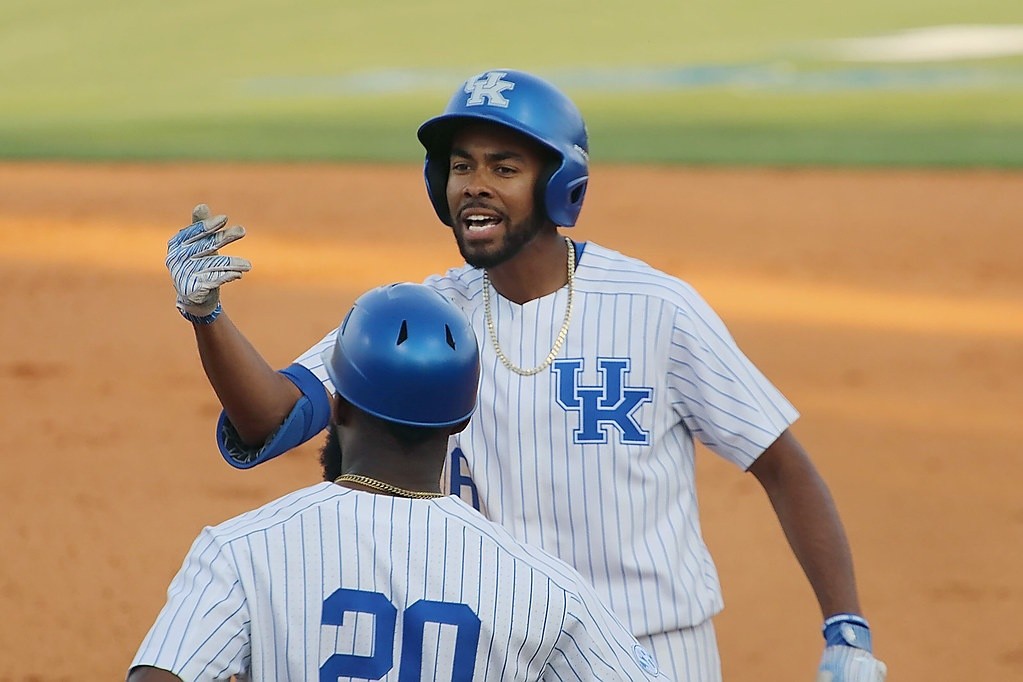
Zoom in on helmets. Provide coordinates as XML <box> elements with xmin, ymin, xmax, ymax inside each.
<box><xmin>319</xmin><ymin>281</ymin><xmax>480</xmax><ymax>425</ymax></box>
<box><xmin>417</xmin><ymin>70</ymin><xmax>589</xmax><ymax>227</ymax></box>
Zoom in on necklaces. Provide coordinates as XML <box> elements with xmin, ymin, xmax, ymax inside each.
<box><xmin>483</xmin><ymin>237</ymin><xmax>575</xmax><ymax>376</ymax></box>
<box><xmin>335</xmin><ymin>474</ymin><xmax>445</xmax><ymax>499</ymax></box>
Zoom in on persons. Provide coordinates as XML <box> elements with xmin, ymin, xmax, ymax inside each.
<box><xmin>126</xmin><ymin>282</ymin><xmax>674</xmax><ymax>682</ymax></box>
<box><xmin>167</xmin><ymin>70</ymin><xmax>893</xmax><ymax>682</ymax></box>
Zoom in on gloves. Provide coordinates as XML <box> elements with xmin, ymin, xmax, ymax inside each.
<box><xmin>165</xmin><ymin>203</ymin><xmax>250</xmax><ymax>323</ymax></box>
<box><xmin>816</xmin><ymin>614</ymin><xmax>886</xmax><ymax>682</ymax></box>
